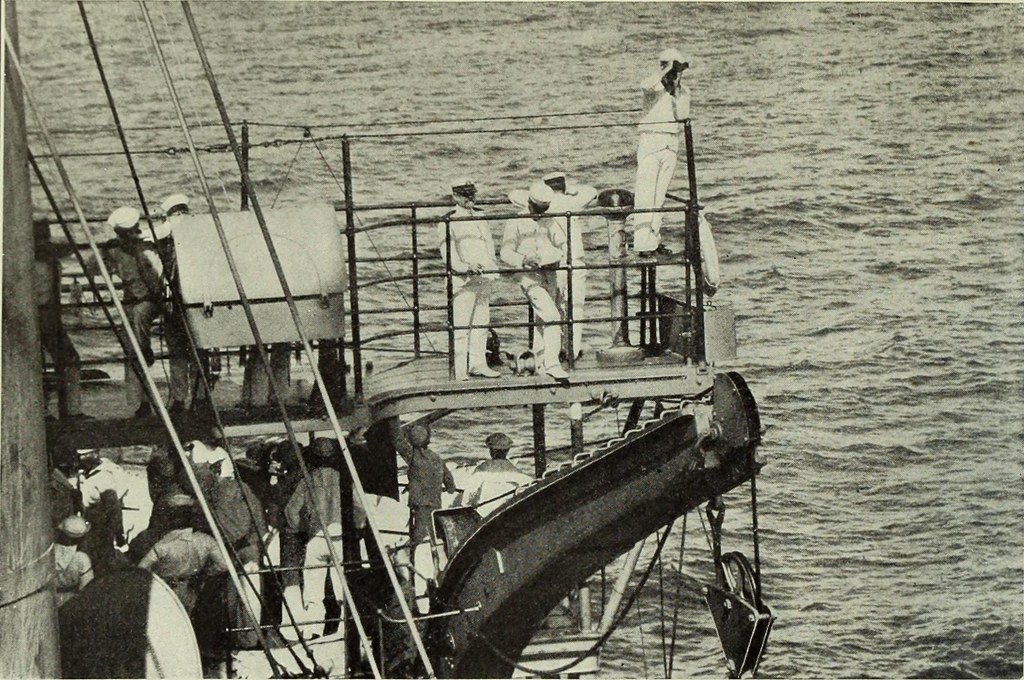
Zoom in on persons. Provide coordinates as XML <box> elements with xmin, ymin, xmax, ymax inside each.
<box><xmin>32</xmin><ymin>193</ymin><xmax>214</xmax><ymax>422</ymax></box>
<box><xmin>52</xmin><ymin>415</ymin><xmax>538</xmax><ymax>650</ymax></box>
<box><xmin>436</xmin><ymin>169</ymin><xmax>600</xmax><ymax>384</ymax></box>
<box><xmin>633</xmin><ymin>47</ymin><xmax>692</xmax><ymax>258</ymax></box>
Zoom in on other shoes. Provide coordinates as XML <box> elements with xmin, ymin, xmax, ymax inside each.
<box><xmin>469</xmin><ymin>364</ymin><xmax>500</xmax><ymax>378</ymax></box>
<box><xmin>189</xmin><ymin>397</ymin><xmax>210</xmax><ymax>417</ymax></box>
<box><xmin>638</xmin><ymin>243</ymin><xmax>672</xmax><ymax>260</ymax></box>
<box><xmin>45</xmin><ymin>413</ymin><xmax>95</xmax><ymax>422</ymax></box>
<box><xmin>135</xmin><ymin>402</ymin><xmax>151</xmax><ymax>417</ymax></box>
<box><xmin>559</xmin><ymin>350</ymin><xmax>583</xmax><ymax>363</ymax></box>
<box><xmin>142</xmin><ymin>346</ymin><xmax>155</xmax><ymax>367</ymax></box>
<box><xmin>455</xmin><ymin>371</ymin><xmax>469</xmax><ymax>381</ymax></box>
<box><xmin>546</xmin><ymin>365</ymin><xmax>570</xmax><ymax>379</ymax></box>
<box><xmin>169</xmin><ymin>398</ymin><xmax>185</xmax><ymax>415</ymax></box>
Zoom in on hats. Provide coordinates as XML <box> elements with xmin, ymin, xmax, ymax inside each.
<box><xmin>167</xmin><ymin>494</ymin><xmax>193</xmax><ymax>508</ymax></box>
<box><xmin>451</xmin><ymin>178</ymin><xmax>477</xmax><ymax>193</ymax></box>
<box><xmin>486</xmin><ymin>433</ymin><xmax>513</xmax><ymax>450</ymax></box>
<box><xmin>529</xmin><ymin>184</ymin><xmax>554</xmax><ymax>205</ymax></box>
<box><xmin>161</xmin><ymin>195</ymin><xmax>189</xmax><ymax>216</ymax></box>
<box><xmin>660</xmin><ymin>49</ymin><xmax>689</xmax><ymax>70</ymax></box>
<box><xmin>409</xmin><ymin>424</ymin><xmax>430</xmax><ymax>448</ymax></box>
<box><xmin>543</xmin><ymin>172</ymin><xmax>565</xmax><ymax>184</ymax></box>
<box><xmin>107</xmin><ymin>207</ymin><xmax>140</xmax><ymax>228</ymax></box>
<box><xmin>58</xmin><ymin>515</ymin><xmax>91</xmax><ymax>538</ymax></box>
<box><xmin>51</xmin><ymin>468</ymin><xmax>74</xmax><ymax>490</ymax></box>
<box><xmin>235</xmin><ymin>437</ymin><xmax>337</xmax><ymax>472</ymax></box>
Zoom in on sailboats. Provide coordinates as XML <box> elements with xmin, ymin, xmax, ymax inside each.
<box><xmin>2</xmin><ymin>1</ymin><xmax>782</xmax><ymax>678</ymax></box>
